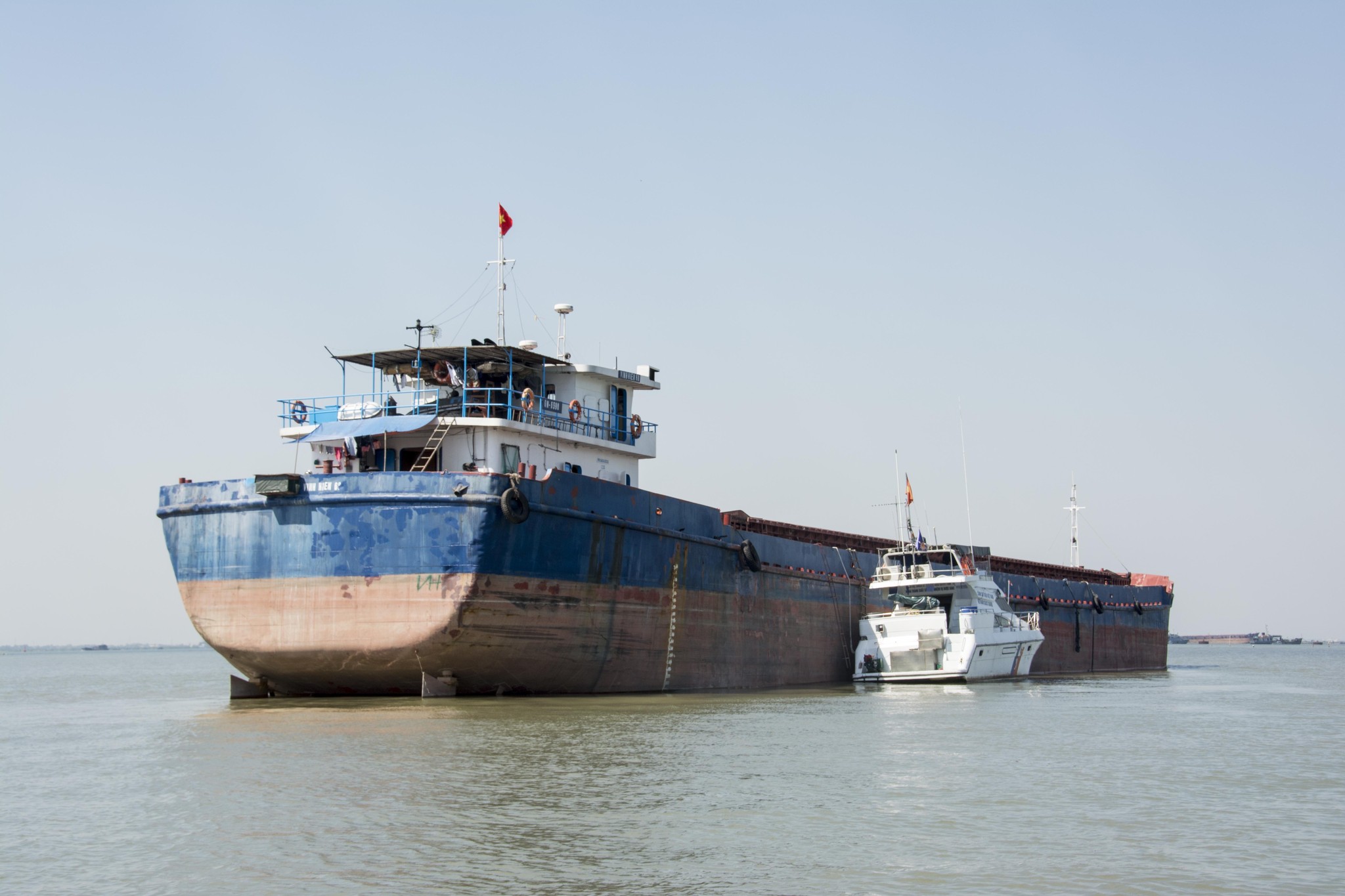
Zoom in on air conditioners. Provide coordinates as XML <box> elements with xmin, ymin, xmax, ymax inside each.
<box><xmin>911</xmin><ymin>564</ymin><xmax>932</xmax><ymax>579</ymax></box>
<box><xmin>876</xmin><ymin>565</ymin><xmax>901</xmax><ymax>582</ymax></box>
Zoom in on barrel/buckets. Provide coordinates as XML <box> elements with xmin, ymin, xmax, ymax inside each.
<box><xmin>960</xmin><ymin>606</ymin><xmax>978</xmax><ymax>613</ymax></box>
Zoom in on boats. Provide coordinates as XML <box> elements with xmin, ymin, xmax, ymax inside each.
<box><xmin>1250</xmin><ymin>633</ymin><xmax>1274</xmax><ymax>644</ymax></box>
<box><xmin>1198</xmin><ymin>641</ymin><xmax>1209</xmax><ymax>644</ymax></box>
<box><xmin>1167</xmin><ymin>634</ymin><xmax>1189</xmax><ymax>644</ymax></box>
<box><xmin>155</xmin><ymin>199</ymin><xmax>1174</xmax><ymax>699</ymax></box>
<box><xmin>1275</xmin><ymin>638</ymin><xmax>1302</xmax><ymax>645</ymax></box>
<box><xmin>1313</xmin><ymin>642</ymin><xmax>1323</xmax><ymax>645</ymax></box>
<box><xmin>848</xmin><ymin>449</ymin><xmax>1046</xmax><ymax>684</ymax></box>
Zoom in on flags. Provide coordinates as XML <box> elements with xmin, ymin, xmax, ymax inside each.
<box><xmin>915</xmin><ymin>529</ymin><xmax>927</xmax><ymax>552</ymax></box>
<box><xmin>499</xmin><ymin>202</ymin><xmax>512</xmax><ymax>236</ymax></box>
<box><xmin>905</xmin><ymin>472</ymin><xmax>913</xmax><ymax>507</ymax></box>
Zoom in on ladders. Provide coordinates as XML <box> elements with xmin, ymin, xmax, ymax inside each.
<box><xmin>409</xmin><ymin>417</ymin><xmax>455</xmax><ymax>472</ymax></box>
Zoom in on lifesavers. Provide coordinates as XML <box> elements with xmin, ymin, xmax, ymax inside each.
<box><xmin>569</xmin><ymin>399</ymin><xmax>582</xmax><ymax>423</ymax></box>
<box><xmin>501</xmin><ymin>488</ymin><xmax>530</xmax><ymax>524</ymax></box>
<box><xmin>1134</xmin><ymin>600</ymin><xmax>1144</xmax><ymax>615</ymax></box>
<box><xmin>291</xmin><ymin>400</ymin><xmax>307</xmax><ymax>423</ymax></box>
<box><xmin>1093</xmin><ymin>596</ymin><xmax>1104</xmax><ymax>614</ymax></box>
<box><xmin>961</xmin><ymin>556</ymin><xmax>974</xmax><ymax>575</ymax></box>
<box><xmin>631</xmin><ymin>414</ymin><xmax>642</xmax><ymax>438</ymax></box>
<box><xmin>521</xmin><ymin>387</ymin><xmax>535</xmax><ymax>412</ymax></box>
<box><xmin>741</xmin><ymin>539</ymin><xmax>761</xmax><ymax>573</ymax></box>
<box><xmin>1039</xmin><ymin>593</ymin><xmax>1049</xmax><ymax>610</ymax></box>
<box><xmin>433</xmin><ymin>360</ymin><xmax>452</xmax><ymax>383</ymax></box>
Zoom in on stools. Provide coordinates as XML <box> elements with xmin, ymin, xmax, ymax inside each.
<box><xmin>468</xmin><ymin>381</ymin><xmax>495</xmax><ymax>417</ymax></box>
